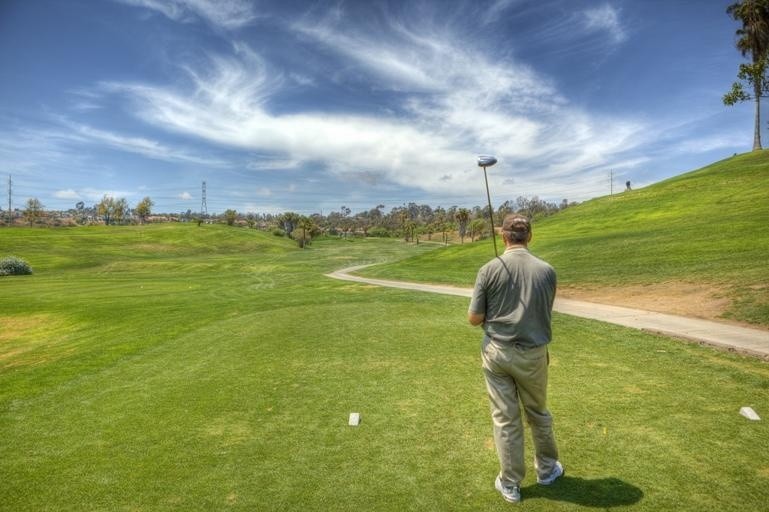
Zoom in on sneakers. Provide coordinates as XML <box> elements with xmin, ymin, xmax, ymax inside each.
<box><xmin>536</xmin><ymin>461</ymin><xmax>563</xmax><ymax>486</ymax></box>
<box><xmin>494</xmin><ymin>475</ymin><xmax>520</xmax><ymax>503</ymax></box>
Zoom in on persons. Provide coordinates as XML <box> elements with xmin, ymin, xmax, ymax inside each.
<box><xmin>468</xmin><ymin>214</ymin><xmax>564</xmax><ymax>504</ymax></box>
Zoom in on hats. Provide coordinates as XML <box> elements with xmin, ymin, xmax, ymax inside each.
<box><xmin>500</xmin><ymin>214</ymin><xmax>530</xmax><ymax>231</ymax></box>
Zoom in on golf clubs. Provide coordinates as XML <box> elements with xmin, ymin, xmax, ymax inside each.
<box><xmin>478</xmin><ymin>157</ymin><xmax>501</xmax><ymax>257</ymax></box>
